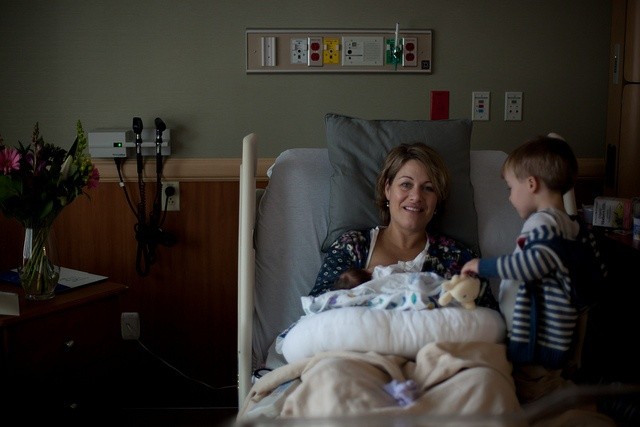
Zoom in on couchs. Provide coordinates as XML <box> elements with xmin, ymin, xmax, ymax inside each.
<box><xmin>239</xmin><ymin>134</ymin><xmax>615</xmax><ymax>426</ymax></box>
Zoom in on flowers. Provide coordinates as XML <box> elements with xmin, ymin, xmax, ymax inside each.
<box><xmin>1</xmin><ymin>120</ymin><xmax>98</xmax><ymax>227</ymax></box>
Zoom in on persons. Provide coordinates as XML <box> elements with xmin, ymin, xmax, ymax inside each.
<box><xmin>336</xmin><ymin>261</ymin><xmax>418</xmax><ymax>289</ymax></box>
<box><xmin>462</xmin><ymin>138</ymin><xmax>609</xmax><ymax>371</ymax></box>
<box><xmin>274</xmin><ymin>143</ymin><xmax>498</xmax><ymax>311</ymax></box>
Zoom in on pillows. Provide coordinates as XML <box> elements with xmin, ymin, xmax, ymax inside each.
<box><xmin>320</xmin><ymin>113</ymin><xmax>478</xmax><ymax>253</ymax></box>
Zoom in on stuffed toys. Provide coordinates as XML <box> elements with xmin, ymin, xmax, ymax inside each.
<box><xmin>439</xmin><ymin>274</ymin><xmax>482</xmax><ymax>312</ymax></box>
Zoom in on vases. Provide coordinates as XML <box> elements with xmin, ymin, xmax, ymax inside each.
<box><xmin>20</xmin><ymin>228</ymin><xmax>61</xmax><ymax>301</ymax></box>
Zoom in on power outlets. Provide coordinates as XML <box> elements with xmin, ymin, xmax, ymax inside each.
<box><xmin>161</xmin><ymin>182</ymin><xmax>180</xmax><ymax>210</ymax></box>
